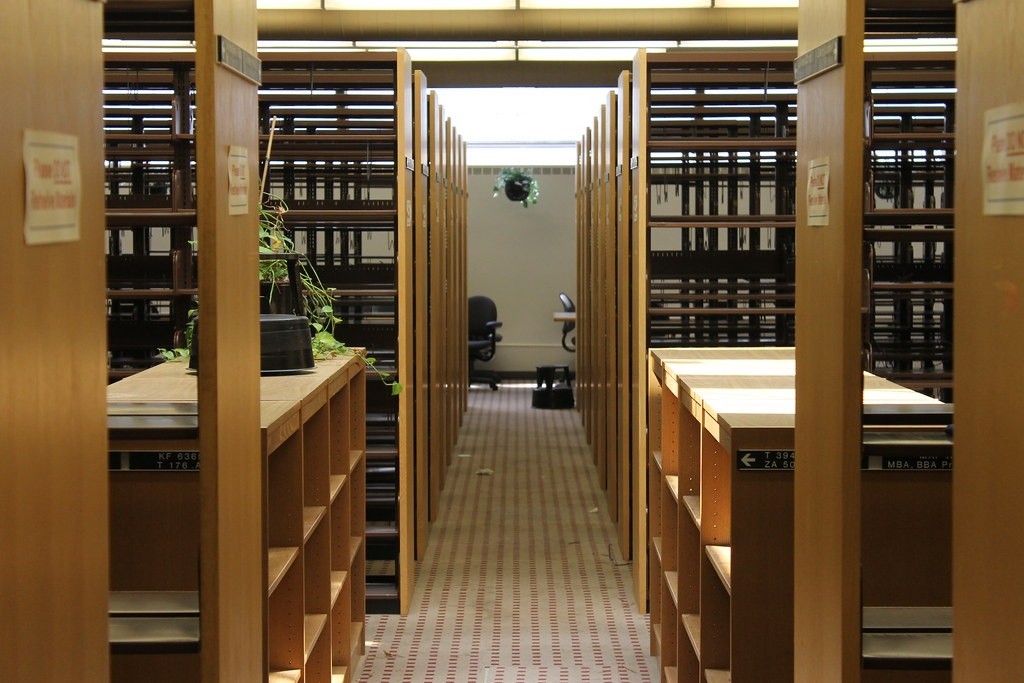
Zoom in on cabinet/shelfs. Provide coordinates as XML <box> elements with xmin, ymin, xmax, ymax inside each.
<box><xmin>573</xmin><ymin>0</ymin><xmax>1024</xmax><ymax>683</ymax></box>
<box><xmin>0</xmin><ymin>0</ymin><xmax>471</xmax><ymax>683</ymax></box>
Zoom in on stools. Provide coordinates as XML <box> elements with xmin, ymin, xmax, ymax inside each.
<box><xmin>536</xmin><ymin>364</ymin><xmax>571</xmax><ymax>401</ymax></box>
<box><xmin>189</xmin><ymin>253</ymin><xmax>315</xmax><ymax>371</ymax></box>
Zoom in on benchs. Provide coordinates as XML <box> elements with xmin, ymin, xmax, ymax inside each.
<box><xmin>553</xmin><ymin>311</ymin><xmax>576</xmax><ymax>323</ymax></box>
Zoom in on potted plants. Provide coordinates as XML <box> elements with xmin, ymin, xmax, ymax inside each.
<box><xmin>493</xmin><ymin>167</ymin><xmax>540</xmax><ymax>208</ymax></box>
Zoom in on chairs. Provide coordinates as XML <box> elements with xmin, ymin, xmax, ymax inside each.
<box><xmin>559</xmin><ymin>292</ymin><xmax>576</xmax><ymax>353</ymax></box>
<box><xmin>468</xmin><ymin>296</ymin><xmax>503</xmax><ymax>391</ymax></box>
